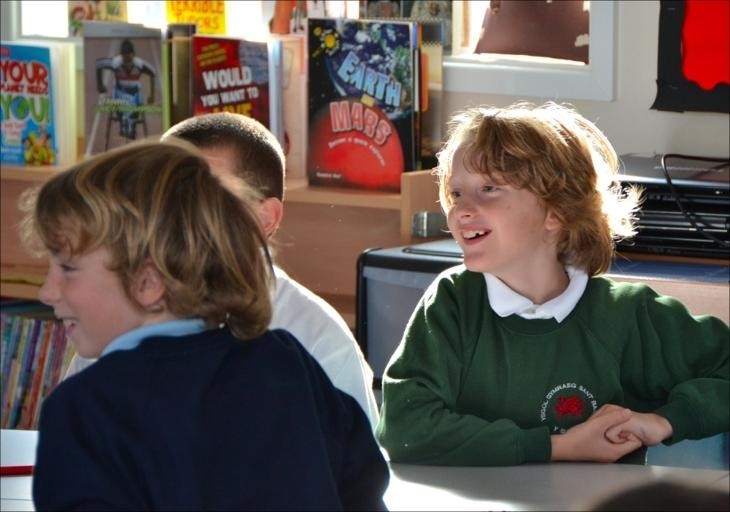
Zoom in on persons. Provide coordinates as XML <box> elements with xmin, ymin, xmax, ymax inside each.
<box><xmin>62</xmin><ymin>114</ymin><xmax>380</xmax><ymax>439</ymax></box>
<box><xmin>20</xmin><ymin>141</ymin><xmax>390</xmax><ymax>511</ymax></box>
<box><xmin>376</xmin><ymin>102</ymin><xmax>730</xmax><ymax>464</ymax></box>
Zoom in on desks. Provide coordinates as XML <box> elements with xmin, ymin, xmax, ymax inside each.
<box><xmin>0</xmin><ymin>460</ymin><xmax>729</xmax><ymax>510</ymax></box>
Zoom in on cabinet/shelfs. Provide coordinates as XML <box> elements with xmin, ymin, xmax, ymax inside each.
<box><xmin>0</xmin><ymin>160</ymin><xmax>445</xmax><ymax>325</ymax></box>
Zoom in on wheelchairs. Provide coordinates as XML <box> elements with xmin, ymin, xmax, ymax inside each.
<box><xmin>102</xmin><ymin>95</ymin><xmax>150</xmax><ymax>154</ymax></box>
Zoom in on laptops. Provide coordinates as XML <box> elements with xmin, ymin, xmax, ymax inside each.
<box><xmin>607</xmin><ymin>152</ymin><xmax>730</xmax><ymax>197</ymax></box>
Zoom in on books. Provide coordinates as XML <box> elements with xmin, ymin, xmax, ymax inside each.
<box><xmin>2</xmin><ymin>302</ymin><xmax>76</xmax><ymax>429</ymax></box>
